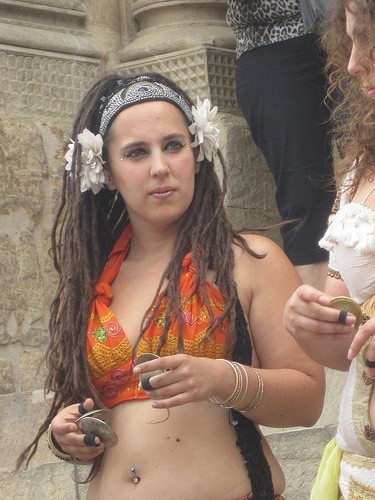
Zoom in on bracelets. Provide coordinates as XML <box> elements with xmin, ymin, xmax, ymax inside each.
<box><xmin>45</xmin><ymin>423</ymin><xmax>82</xmax><ymax>463</ymax></box>
<box><xmin>208</xmin><ymin>358</ymin><xmax>264</xmax><ymax>413</ymax></box>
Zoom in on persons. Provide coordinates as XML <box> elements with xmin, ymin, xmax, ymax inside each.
<box><xmin>15</xmin><ymin>72</ymin><xmax>326</xmax><ymax>500</ymax></box>
<box><xmin>283</xmin><ymin>0</ymin><xmax>375</xmax><ymax>500</ymax></box>
<box><xmin>225</xmin><ymin>0</ymin><xmax>338</xmax><ymax>292</ymax></box>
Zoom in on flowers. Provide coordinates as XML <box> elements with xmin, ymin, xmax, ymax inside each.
<box><xmin>65</xmin><ymin>126</ymin><xmax>108</xmax><ymax>196</ymax></box>
<box><xmin>190</xmin><ymin>95</ymin><xmax>223</xmax><ymax>162</ymax></box>
